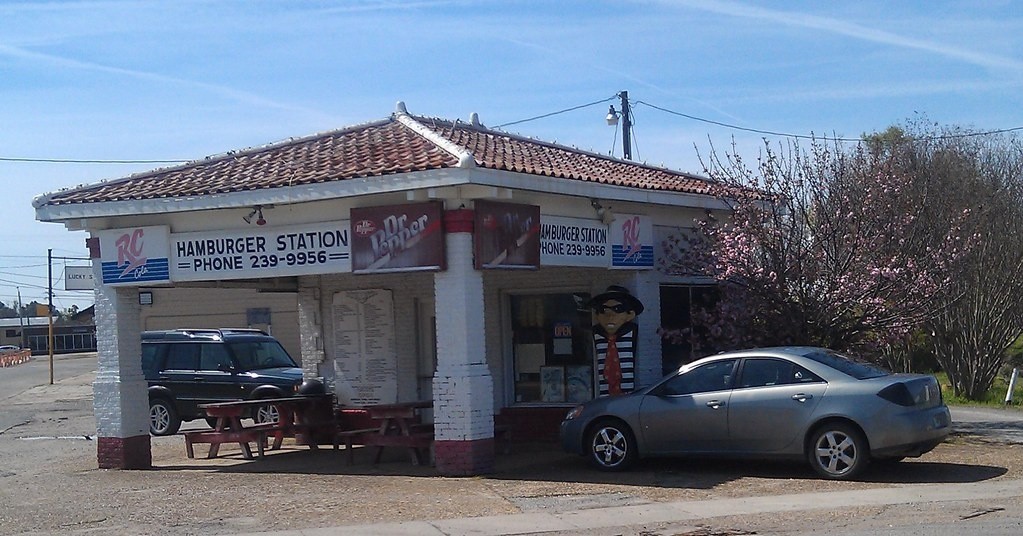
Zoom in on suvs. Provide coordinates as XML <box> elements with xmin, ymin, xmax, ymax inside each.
<box><xmin>141</xmin><ymin>325</ymin><xmax>304</xmax><ymax>437</ymax></box>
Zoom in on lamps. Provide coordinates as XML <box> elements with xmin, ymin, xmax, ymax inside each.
<box><xmin>243</xmin><ymin>206</ymin><xmax>266</xmax><ymax>226</ymax></box>
<box><xmin>705</xmin><ymin>210</ymin><xmax>719</xmax><ymax>221</ymax></box>
<box><xmin>590</xmin><ymin>200</ymin><xmax>607</xmax><ymax>213</ymax></box>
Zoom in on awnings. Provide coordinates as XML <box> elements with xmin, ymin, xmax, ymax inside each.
<box><xmin>71</xmin><ymin>305</ymin><xmax>95</xmax><ymax>320</ymax></box>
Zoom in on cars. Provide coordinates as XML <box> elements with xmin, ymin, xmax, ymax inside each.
<box><xmin>0</xmin><ymin>344</ymin><xmax>31</xmax><ymax>354</ymax></box>
<box><xmin>558</xmin><ymin>345</ymin><xmax>952</xmax><ymax>481</ymax></box>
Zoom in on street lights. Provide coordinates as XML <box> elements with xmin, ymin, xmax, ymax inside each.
<box><xmin>605</xmin><ymin>89</ymin><xmax>634</xmax><ymax>160</ymax></box>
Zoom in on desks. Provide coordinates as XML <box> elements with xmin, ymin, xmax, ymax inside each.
<box><xmin>194</xmin><ymin>395</ymin><xmax>334</xmax><ymax>461</ymax></box>
<box><xmin>358</xmin><ymin>399</ymin><xmax>435</xmax><ymax>467</ymax></box>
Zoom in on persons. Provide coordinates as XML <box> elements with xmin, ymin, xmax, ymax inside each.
<box><xmin>588</xmin><ymin>285</ymin><xmax>644</xmax><ymax>396</ymax></box>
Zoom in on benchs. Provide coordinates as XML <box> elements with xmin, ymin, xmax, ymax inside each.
<box><xmin>177</xmin><ymin>421</ymin><xmax>511</xmax><ymax>468</ymax></box>
